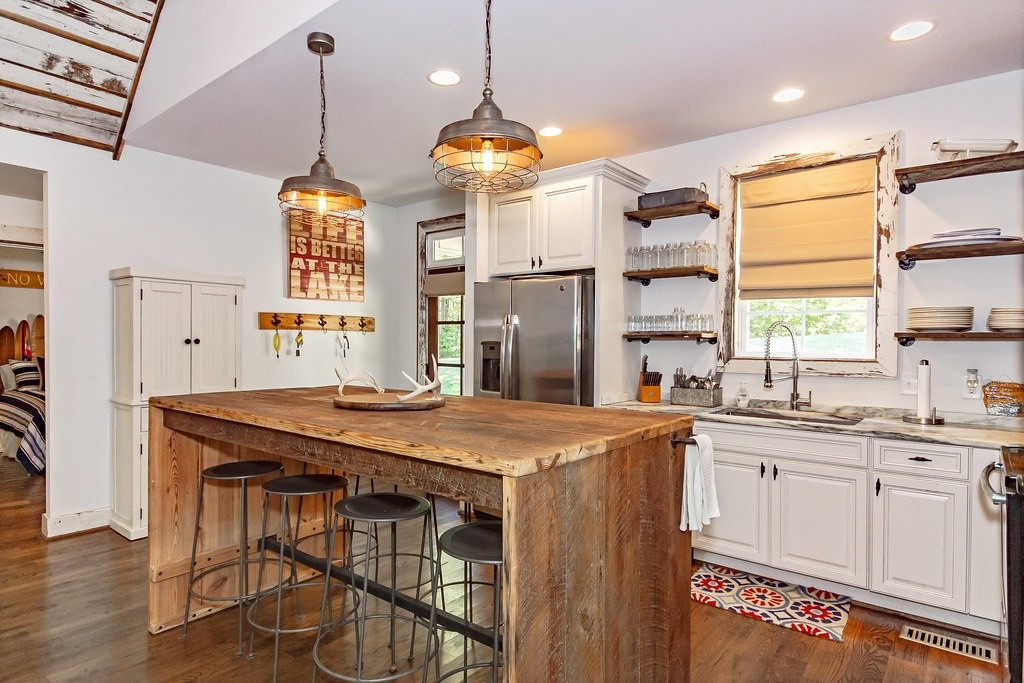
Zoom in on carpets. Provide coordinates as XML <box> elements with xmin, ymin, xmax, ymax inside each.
<box><xmin>688</xmin><ymin>562</ymin><xmax>849</xmax><ymax>643</ymax></box>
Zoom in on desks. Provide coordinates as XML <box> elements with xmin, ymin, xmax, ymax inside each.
<box><xmin>147</xmin><ymin>382</ymin><xmax>696</xmax><ymax>683</ymax></box>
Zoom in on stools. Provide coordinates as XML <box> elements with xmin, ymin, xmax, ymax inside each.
<box><xmin>310</xmin><ymin>493</ymin><xmax>439</xmax><ymax>682</ymax></box>
<box><xmin>423</xmin><ymin>519</ymin><xmax>505</xmax><ymax>682</ymax></box>
<box><xmin>179</xmin><ymin>460</ymin><xmax>304</xmax><ymax>657</ymax></box>
<box><xmin>242</xmin><ymin>473</ymin><xmax>365</xmax><ymax>681</ymax></box>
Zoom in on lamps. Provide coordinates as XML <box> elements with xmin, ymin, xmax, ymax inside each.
<box><xmin>428</xmin><ymin>0</ymin><xmax>544</xmax><ymax>194</ymax></box>
<box><xmin>277</xmin><ymin>31</ymin><xmax>367</xmax><ymax>230</ymax></box>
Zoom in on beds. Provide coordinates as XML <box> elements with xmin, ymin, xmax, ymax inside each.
<box><xmin>0</xmin><ymin>365</ymin><xmax>46</xmax><ymax>477</ymax></box>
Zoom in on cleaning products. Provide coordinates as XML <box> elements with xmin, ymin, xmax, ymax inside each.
<box><xmin>735</xmin><ymin>381</ymin><xmax>750</xmax><ymax>408</ymax></box>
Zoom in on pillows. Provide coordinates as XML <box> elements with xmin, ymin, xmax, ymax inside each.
<box><xmin>8</xmin><ymin>358</ymin><xmax>40</xmax><ymax>391</ymax></box>
<box><xmin>35</xmin><ymin>356</ymin><xmax>45</xmax><ymax>393</ymax></box>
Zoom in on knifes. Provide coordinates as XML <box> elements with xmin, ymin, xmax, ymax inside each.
<box><xmin>642</xmin><ymin>356</ymin><xmax>662</xmax><ymax>386</ymax></box>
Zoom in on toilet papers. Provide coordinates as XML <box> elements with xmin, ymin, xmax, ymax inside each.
<box><xmin>917</xmin><ymin>363</ymin><xmax>931</xmax><ymax>418</ymax></box>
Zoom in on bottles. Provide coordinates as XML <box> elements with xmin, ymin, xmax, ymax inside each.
<box><xmin>628</xmin><ymin>306</ymin><xmax>715</xmax><ymax>333</ymax></box>
<box><xmin>631</xmin><ymin>240</ymin><xmax>718</xmax><ymax>270</ymax></box>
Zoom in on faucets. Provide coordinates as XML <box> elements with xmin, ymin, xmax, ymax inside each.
<box><xmin>763</xmin><ymin>320</ymin><xmax>812</xmax><ymax>411</ymax></box>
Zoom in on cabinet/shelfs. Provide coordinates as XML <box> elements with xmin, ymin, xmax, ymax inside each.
<box><xmin>487</xmin><ymin>171</ymin><xmax>597</xmax><ymax>278</ymax></box>
<box><xmin>687</xmin><ymin>416</ymin><xmax>1010</xmax><ymax>641</ymax></box>
<box><xmin>618</xmin><ymin>201</ymin><xmax>721</xmax><ymax>341</ymax></box>
<box><xmin>894</xmin><ymin>150</ymin><xmax>1024</xmax><ymax>345</ymax></box>
<box><xmin>109</xmin><ymin>265</ymin><xmax>244</xmax><ymax>540</ymax></box>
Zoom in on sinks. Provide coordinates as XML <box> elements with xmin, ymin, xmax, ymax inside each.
<box><xmin>710</xmin><ymin>406</ymin><xmax>864</xmax><ymax>425</ymax></box>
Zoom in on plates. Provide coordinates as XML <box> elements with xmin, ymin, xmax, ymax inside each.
<box><xmin>907</xmin><ymin>227</ymin><xmax>1022</xmax><ymax>248</ymax></box>
<box><xmin>905</xmin><ymin>306</ymin><xmax>1024</xmax><ymax>334</ymax></box>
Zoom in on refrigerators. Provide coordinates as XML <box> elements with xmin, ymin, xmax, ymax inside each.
<box><xmin>472</xmin><ymin>275</ymin><xmax>593</xmax><ymax>408</ymax></box>
